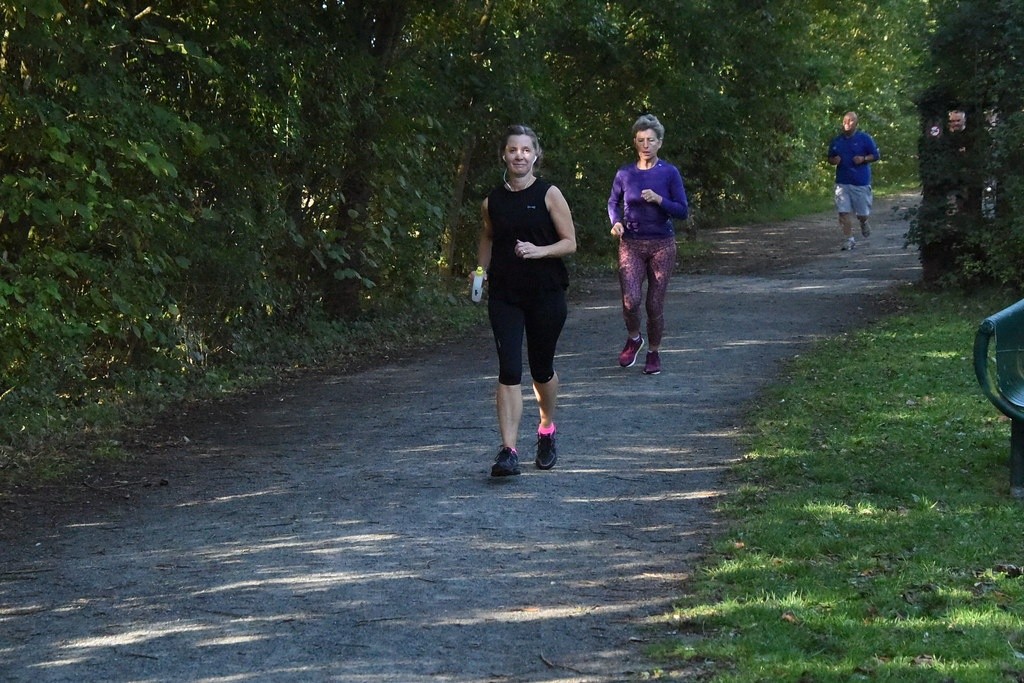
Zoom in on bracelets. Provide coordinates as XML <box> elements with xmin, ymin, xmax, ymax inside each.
<box><xmin>864</xmin><ymin>156</ymin><xmax>867</xmax><ymax>162</ymax></box>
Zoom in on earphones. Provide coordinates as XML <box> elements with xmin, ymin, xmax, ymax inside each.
<box><xmin>503</xmin><ymin>155</ymin><xmax>507</xmax><ymax>162</ymax></box>
<box><xmin>533</xmin><ymin>155</ymin><xmax>537</xmax><ymax>163</ymax></box>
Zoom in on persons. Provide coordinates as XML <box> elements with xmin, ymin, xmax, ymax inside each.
<box><xmin>468</xmin><ymin>124</ymin><xmax>577</xmax><ymax>478</ymax></box>
<box><xmin>827</xmin><ymin>111</ymin><xmax>880</xmax><ymax>251</ymax></box>
<box><xmin>948</xmin><ymin>110</ymin><xmax>967</xmax><ymax>136</ymax></box>
<box><xmin>607</xmin><ymin>114</ymin><xmax>688</xmax><ymax>375</ymax></box>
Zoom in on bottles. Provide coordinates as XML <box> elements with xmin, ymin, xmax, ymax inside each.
<box><xmin>471</xmin><ymin>266</ymin><xmax>484</xmax><ymax>302</ymax></box>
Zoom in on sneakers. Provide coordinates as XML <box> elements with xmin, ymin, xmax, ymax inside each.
<box><xmin>861</xmin><ymin>220</ymin><xmax>871</xmax><ymax>237</ymax></box>
<box><xmin>642</xmin><ymin>350</ymin><xmax>661</xmax><ymax>375</ymax></box>
<box><xmin>533</xmin><ymin>421</ymin><xmax>557</xmax><ymax>470</ymax></box>
<box><xmin>618</xmin><ymin>331</ymin><xmax>645</xmax><ymax>368</ymax></box>
<box><xmin>839</xmin><ymin>238</ymin><xmax>856</xmax><ymax>250</ymax></box>
<box><xmin>491</xmin><ymin>445</ymin><xmax>522</xmax><ymax>477</ymax></box>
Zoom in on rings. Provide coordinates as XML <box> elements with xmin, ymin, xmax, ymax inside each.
<box><xmin>522</xmin><ymin>250</ymin><xmax>525</xmax><ymax>254</ymax></box>
